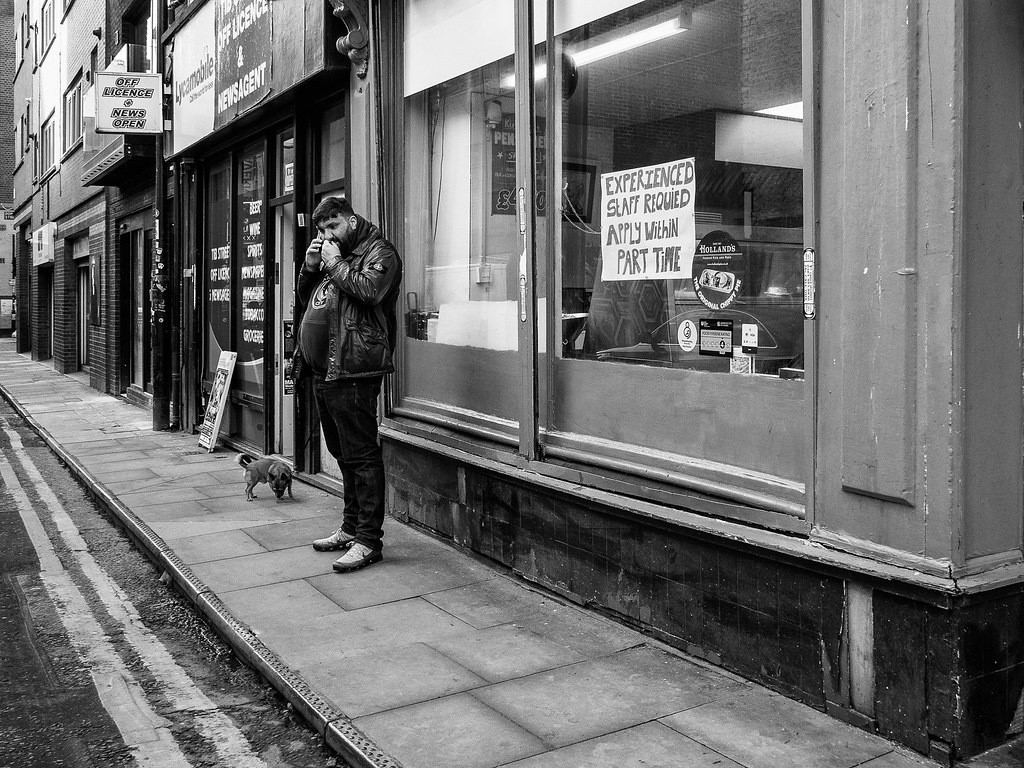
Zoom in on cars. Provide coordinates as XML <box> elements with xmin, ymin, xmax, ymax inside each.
<box><xmin>596</xmin><ymin>307</ymin><xmax>805</xmax><ymax>378</ymax></box>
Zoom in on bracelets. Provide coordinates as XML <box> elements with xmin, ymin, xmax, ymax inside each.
<box><xmin>332</xmin><ymin>253</ymin><xmax>341</xmax><ymax>259</ymax></box>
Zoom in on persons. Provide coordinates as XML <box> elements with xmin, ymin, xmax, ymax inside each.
<box><xmin>293</xmin><ymin>195</ymin><xmax>403</xmax><ymax>571</ymax></box>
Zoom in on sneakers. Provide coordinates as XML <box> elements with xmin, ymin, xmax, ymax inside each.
<box><xmin>312</xmin><ymin>527</ymin><xmax>357</xmax><ymax>552</ymax></box>
<box><xmin>332</xmin><ymin>540</ymin><xmax>383</xmax><ymax>573</ymax></box>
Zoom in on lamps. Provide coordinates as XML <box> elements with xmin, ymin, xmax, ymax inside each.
<box><xmin>499</xmin><ymin>4</ymin><xmax>693</xmax><ymax>91</ymax></box>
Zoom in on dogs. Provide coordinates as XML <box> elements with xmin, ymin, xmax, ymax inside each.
<box><xmin>236</xmin><ymin>453</ymin><xmax>293</xmax><ymax>502</ymax></box>
